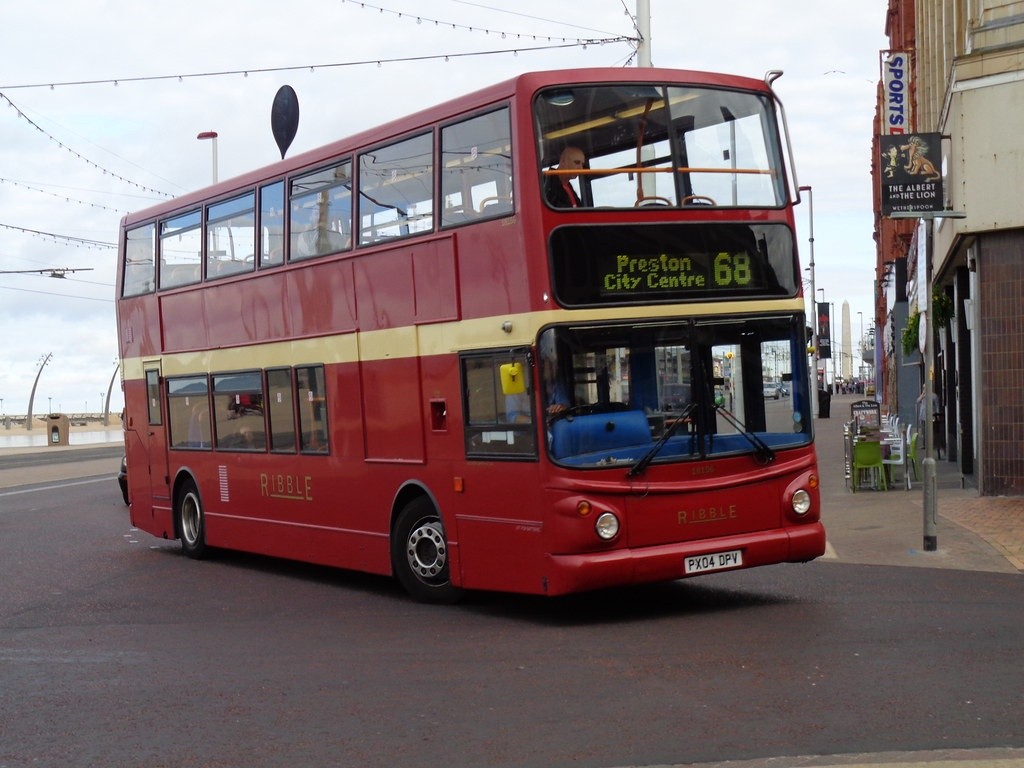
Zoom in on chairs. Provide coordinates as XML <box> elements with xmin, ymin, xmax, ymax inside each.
<box><xmin>167</xmin><ymin>195</ymin><xmax>716</xmax><ymax>283</ymax></box>
<box><xmin>843</xmin><ymin>413</ymin><xmax>919</xmax><ymax>491</ymax></box>
<box><xmin>188</xmin><ymin>403</ymin><xmax>238</xmax><ymax>450</ymax></box>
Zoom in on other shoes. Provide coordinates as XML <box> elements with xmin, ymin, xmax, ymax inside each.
<box><xmin>919</xmin><ymin>445</ymin><xmax>925</xmax><ymax>449</ymax></box>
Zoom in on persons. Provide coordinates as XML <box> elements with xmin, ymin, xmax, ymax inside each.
<box><xmin>545</xmin><ymin>147</ymin><xmax>585</xmax><ymax>208</ymax></box>
<box><xmin>836</xmin><ymin>384</ymin><xmax>839</xmax><ymax>394</ymax></box>
<box><xmin>230</xmin><ymin>422</ymin><xmax>265</xmax><ymax>449</ymax></box>
<box><xmin>297</xmin><ymin>204</ymin><xmax>344</xmax><ymax>257</ymax></box>
<box><xmin>917</xmin><ymin>384</ymin><xmax>940</xmax><ymax>449</ymax></box>
<box><xmin>842</xmin><ymin>381</ymin><xmax>864</xmax><ymax>394</ymax></box>
<box><xmin>505</xmin><ymin>347</ymin><xmax>570</xmax><ymax>423</ymax></box>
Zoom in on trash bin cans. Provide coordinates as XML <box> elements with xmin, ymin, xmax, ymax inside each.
<box><xmin>818</xmin><ymin>389</ymin><xmax>831</xmax><ymax>417</ymax></box>
<box><xmin>47</xmin><ymin>414</ymin><xmax>70</xmax><ymax>446</ymax></box>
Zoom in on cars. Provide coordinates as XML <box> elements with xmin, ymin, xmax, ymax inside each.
<box><xmin>763</xmin><ymin>381</ymin><xmax>790</xmax><ymax>400</ymax></box>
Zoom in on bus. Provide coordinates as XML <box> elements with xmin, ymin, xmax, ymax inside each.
<box><xmin>113</xmin><ymin>65</ymin><xmax>830</xmax><ymax>607</ymax></box>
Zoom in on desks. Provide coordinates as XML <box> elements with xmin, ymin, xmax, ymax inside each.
<box><xmin>852</xmin><ymin>438</ymin><xmax>894</xmax><ymax>489</ymax></box>
<box><xmin>855</xmin><ymin>434</ymin><xmax>893</xmax><ymax>472</ymax></box>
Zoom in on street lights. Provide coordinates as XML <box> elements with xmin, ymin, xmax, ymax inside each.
<box><xmin>827</xmin><ymin>302</ymin><xmax>837</xmax><ymax>395</ymax></box>
<box><xmin>817</xmin><ymin>288</ymin><xmax>828</xmax><ymax>394</ymax></box>
<box><xmin>858</xmin><ymin>311</ymin><xmax>863</xmax><ymax>385</ymax></box>
<box><xmin>197</xmin><ymin>131</ymin><xmax>221</xmax><ymax>185</ymax></box>
